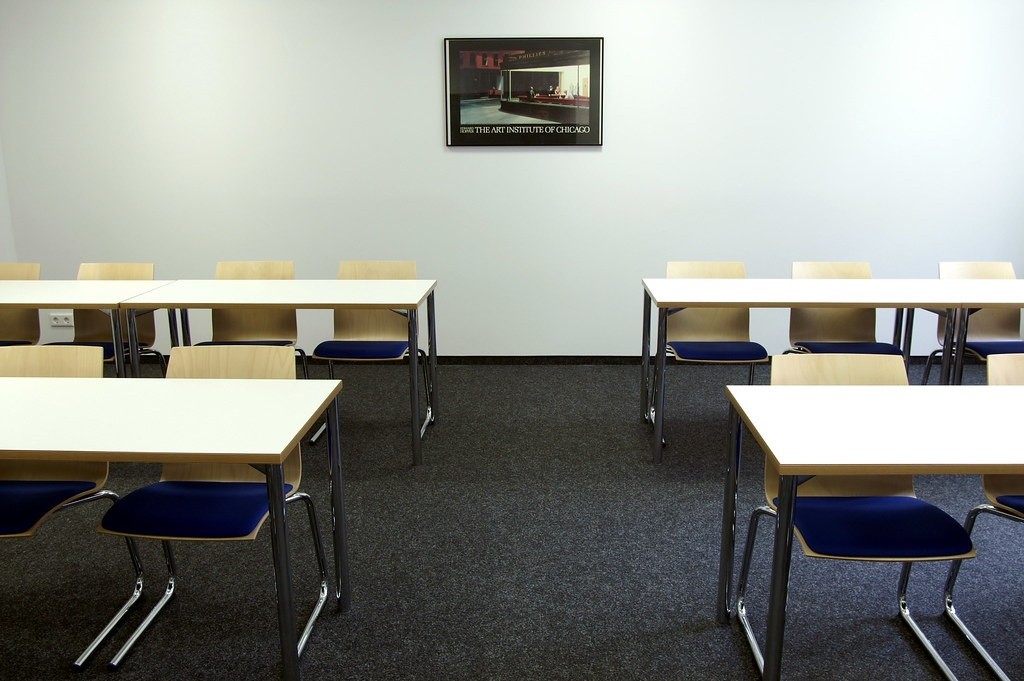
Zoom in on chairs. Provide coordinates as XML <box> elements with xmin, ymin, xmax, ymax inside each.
<box><xmin>194</xmin><ymin>260</ymin><xmax>309</xmax><ymax>379</ymax></box>
<box><xmin>0</xmin><ymin>261</ymin><xmax>41</xmax><ymax>346</ymax></box>
<box><xmin>308</xmin><ymin>259</ymin><xmax>432</xmax><ymax>446</ymax></box>
<box><xmin>94</xmin><ymin>346</ymin><xmax>330</xmax><ymax>675</ymax></box>
<box><xmin>0</xmin><ymin>344</ymin><xmax>146</xmax><ymax>672</ymax></box>
<box><xmin>735</xmin><ymin>352</ymin><xmax>976</xmax><ymax>681</ymax></box>
<box><xmin>921</xmin><ymin>261</ymin><xmax>1024</xmax><ymax>385</ymax></box>
<box><xmin>42</xmin><ymin>263</ymin><xmax>168</xmax><ymax>378</ymax></box>
<box><xmin>782</xmin><ymin>261</ymin><xmax>907</xmax><ymax>362</ymax></box>
<box><xmin>943</xmin><ymin>352</ymin><xmax>1024</xmax><ymax>681</ymax></box>
<box><xmin>647</xmin><ymin>261</ymin><xmax>769</xmax><ymax>449</ymax></box>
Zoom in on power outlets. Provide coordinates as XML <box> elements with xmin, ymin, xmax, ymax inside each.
<box><xmin>50</xmin><ymin>314</ymin><xmax>74</xmax><ymax>327</ymax></box>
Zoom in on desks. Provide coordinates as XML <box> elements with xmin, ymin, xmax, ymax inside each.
<box><xmin>0</xmin><ymin>376</ymin><xmax>351</xmax><ymax>681</ymax></box>
<box><xmin>119</xmin><ymin>279</ymin><xmax>439</xmax><ymax>466</ymax></box>
<box><xmin>715</xmin><ymin>385</ymin><xmax>1024</xmax><ymax>681</ymax></box>
<box><xmin>0</xmin><ymin>280</ymin><xmax>179</xmax><ymax>378</ymax></box>
<box><xmin>640</xmin><ymin>278</ymin><xmax>1024</xmax><ymax>466</ymax></box>
